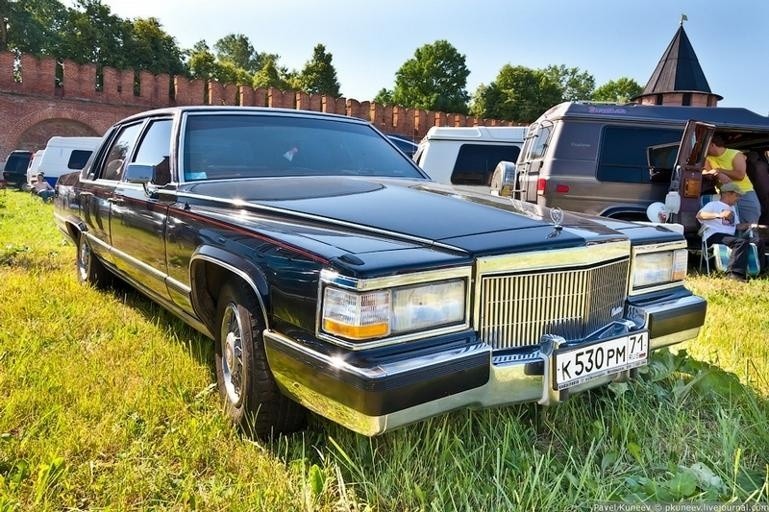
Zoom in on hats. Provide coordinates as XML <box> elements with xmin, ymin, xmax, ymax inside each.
<box><xmin>720</xmin><ymin>183</ymin><xmax>745</xmax><ymax>195</ymax></box>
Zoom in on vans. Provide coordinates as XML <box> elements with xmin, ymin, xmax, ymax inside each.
<box><xmin>412</xmin><ymin>125</ymin><xmax>532</xmax><ymax>194</ymax></box>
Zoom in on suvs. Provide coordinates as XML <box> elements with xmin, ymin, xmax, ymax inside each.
<box><xmin>24</xmin><ymin>135</ymin><xmax>103</xmax><ymax>187</ymax></box>
<box><xmin>3</xmin><ymin>150</ymin><xmax>33</xmax><ymax>192</ymax></box>
<box><xmin>490</xmin><ymin>100</ymin><xmax>768</xmax><ymax>252</ymax></box>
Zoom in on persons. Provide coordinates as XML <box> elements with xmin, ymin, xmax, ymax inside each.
<box><xmin>702</xmin><ymin>136</ymin><xmax>762</xmax><ymax>244</ymax></box>
<box><xmin>32</xmin><ymin>173</ymin><xmax>55</xmax><ymax>202</ymax></box>
<box><xmin>696</xmin><ymin>183</ymin><xmax>751</xmax><ymax>282</ymax></box>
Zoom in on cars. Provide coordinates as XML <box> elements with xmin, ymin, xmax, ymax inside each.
<box><xmin>386</xmin><ymin>133</ymin><xmax>418</xmax><ymax>159</ymax></box>
<box><xmin>53</xmin><ymin>105</ymin><xmax>707</xmax><ymax>441</ymax></box>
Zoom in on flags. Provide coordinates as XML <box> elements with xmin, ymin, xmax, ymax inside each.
<box><xmin>682</xmin><ymin>15</ymin><xmax>688</xmax><ymax>21</ymax></box>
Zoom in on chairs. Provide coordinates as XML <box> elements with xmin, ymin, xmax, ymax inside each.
<box><xmin>699</xmin><ymin>195</ymin><xmax>760</xmax><ymax>278</ymax></box>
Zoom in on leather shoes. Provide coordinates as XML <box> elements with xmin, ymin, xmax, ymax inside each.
<box><xmin>726</xmin><ymin>273</ymin><xmax>745</xmax><ymax>283</ymax></box>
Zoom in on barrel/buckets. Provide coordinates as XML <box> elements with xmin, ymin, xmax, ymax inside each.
<box><xmin>646</xmin><ymin>201</ymin><xmax>670</xmax><ymax>225</ymax></box>
<box><xmin>665</xmin><ymin>191</ymin><xmax>682</xmax><ymax>214</ymax></box>
